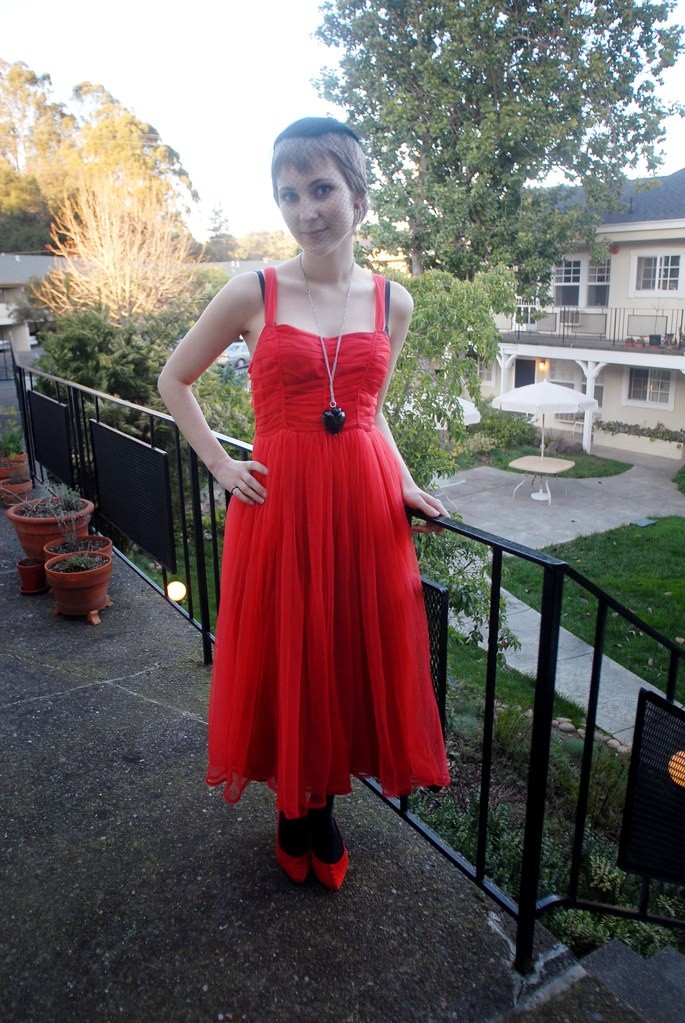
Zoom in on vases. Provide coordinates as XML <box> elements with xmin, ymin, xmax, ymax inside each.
<box><xmin>15</xmin><ymin>558</ymin><xmax>50</xmax><ymax>595</ymax></box>
<box><xmin>649</xmin><ymin>333</ymin><xmax>662</xmax><ymax>344</ymax></box>
<box><xmin>0</xmin><ymin>478</ymin><xmax>33</xmax><ymax>509</ymax></box>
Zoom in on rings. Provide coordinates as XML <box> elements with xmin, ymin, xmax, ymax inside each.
<box><xmin>231</xmin><ymin>486</ymin><xmax>239</xmax><ymax>495</ymax></box>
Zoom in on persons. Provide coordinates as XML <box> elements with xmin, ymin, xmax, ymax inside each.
<box><xmin>156</xmin><ymin>115</ymin><xmax>451</xmax><ymax>893</ymax></box>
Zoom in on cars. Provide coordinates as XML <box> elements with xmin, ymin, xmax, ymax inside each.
<box><xmin>214</xmin><ymin>342</ymin><xmax>251</xmax><ymax>369</ymax></box>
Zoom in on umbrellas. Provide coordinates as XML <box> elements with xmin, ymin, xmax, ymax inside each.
<box><xmin>488</xmin><ymin>379</ymin><xmax>598</xmax><ymax>458</ymax></box>
<box><xmin>386</xmin><ymin>388</ymin><xmax>482</xmax><ymax>432</ymax></box>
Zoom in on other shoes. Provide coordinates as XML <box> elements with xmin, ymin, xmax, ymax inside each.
<box><xmin>309</xmin><ymin>813</ymin><xmax>349</xmax><ymax>890</ymax></box>
<box><xmin>275</xmin><ymin>808</ymin><xmax>307</xmax><ymax>880</ymax></box>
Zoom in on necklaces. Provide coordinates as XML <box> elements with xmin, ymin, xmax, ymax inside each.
<box><xmin>298</xmin><ymin>253</ymin><xmax>356</xmax><ymax>435</ymax></box>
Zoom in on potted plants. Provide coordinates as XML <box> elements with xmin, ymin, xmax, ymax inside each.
<box><xmin>635</xmin><ymin>339</ymin><xmax>645</xmax><ymax>348</ymax></box>
<box><xmin>43</xmin><ymin>518</ymin><xmax>112</xmax><ymax>563</ymax></box>
<box><xmin>0</xmin><ymin>431</ymin><xmax>28</xmax><ymax>480</ymax></box>
<box><xmin>625</xmin><ymin>338</ymin><xmax>634</xmax><ymax>347</ymax></box>
<box><xmin>45</xmin><ymin>552</ymin><xmax>112</xmax><ymax>625</ymax></box>
<box><xmin>0</xmin><ymin>405</ymin><xmax>26</xmax><ymax>460</ymax></box>
<box><xmin>6</xmin><ymin>478</ymin><xmax>94</xmax><ymax>559</ymax></box>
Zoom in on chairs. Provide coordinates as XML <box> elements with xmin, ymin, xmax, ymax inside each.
<box><xmin>573</xmin><ymin>415</ymin><xmax>596</xmax><ymax>436</ymax></box>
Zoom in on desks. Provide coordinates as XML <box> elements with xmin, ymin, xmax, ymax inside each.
<box><xmin>429</xmin><ymin>476</ymin><xmax>465</xmax><ymax>510</ymax></box>
<box><xmin>508</xmin><ymin>456</ymin><xmax>575</xmax><ymax>505</ymax></box>
